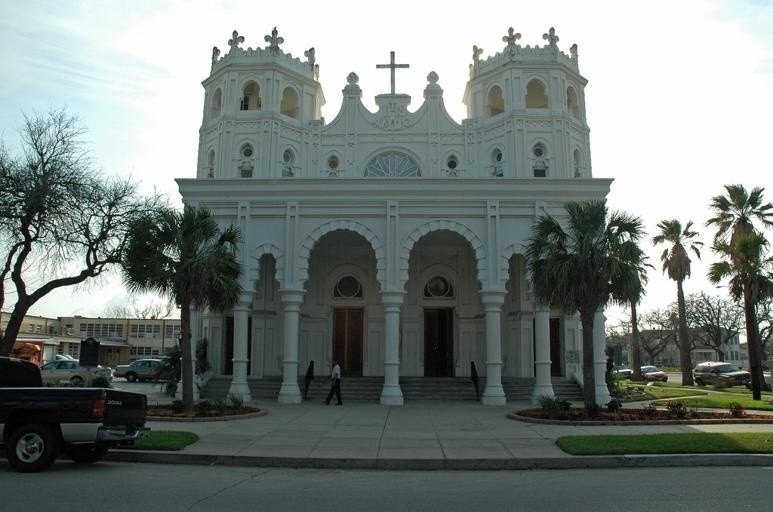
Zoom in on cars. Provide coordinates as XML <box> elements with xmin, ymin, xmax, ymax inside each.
<box><xmin>39</xmin><ymin>354</ymin><xmax>181</xmax><ymax>387</ymax></box>
<box><xmin>693</xmin><ymin>361</ymin><xmax>751</xmax><ymax>388</ymax></box>
<box><xmin>610</xmin><ymin>365</ymin><xmax>668</xmax><ymax>382</ymax></box>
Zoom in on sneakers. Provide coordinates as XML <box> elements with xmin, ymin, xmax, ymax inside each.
<box><xmin>322</xmin><ymin>400</ymin><xmax>343</xmax><ymax>406</ymax></box>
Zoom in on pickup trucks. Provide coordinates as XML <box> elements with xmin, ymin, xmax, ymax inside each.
<box><xmin>0</xmin><ymin>356</ymin><xmax>147</xmax><ymax>474</ymax></box>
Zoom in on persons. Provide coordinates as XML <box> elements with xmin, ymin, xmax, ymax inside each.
<box><xmin>321</xmin><ymin>359</ymin><xmax>343</xmax><ymax>406</ymax></box>
